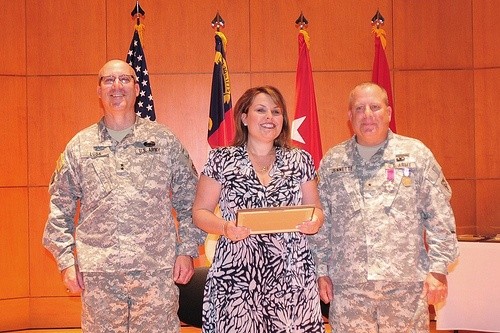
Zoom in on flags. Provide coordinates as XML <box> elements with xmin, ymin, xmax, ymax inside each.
<box><xmin>208</xmin><ymin>31</ymin><xmax>237</xmax><ymax>151</ymax></box>
<box><xmin>290</xmin><ymin>29</ymin><xmax>323</xmax><ymax>171</ymax></box>
<box><xmin>371</xmin><ymin>22</ymin><xmax>397</xmax><ymax>135</ymax></box>
<box><xmin>124</xmin><ymin>23</ymin><xmax>157</xmax><ymax>123</ymax></box>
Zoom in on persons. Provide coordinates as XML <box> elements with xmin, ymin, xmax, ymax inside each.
<box><xmin>190</xmin><ymin>84</ymin><xmax>326</xmax><ymax>333</ymax></box>
<box><xmin>42</xmin><ymin>59</ymin><xmax>207</xmax><ymax>333</ymax></box>
<box><xmin>313</xmin><ymin>80</ymin><xmax>460</xmax><ymax>333</ymax></box>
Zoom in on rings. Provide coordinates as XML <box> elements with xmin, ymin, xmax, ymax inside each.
<box><xmin>441</xmin><ymin>295</ymin><xmax>445</xmax><ymax>298</ymax></box>
<box><xmin>236</xmin><ymin>235</ymin><xmax>238</xmax><ymax>239</ymax></box>
<box><xmin>66</xmin><ymin>289</ymin><xmax>70</xmax><ymax>293</ymax></box>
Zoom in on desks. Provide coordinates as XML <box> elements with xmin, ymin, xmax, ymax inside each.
<box><xmin>436</xmin><ymin>238</ymin><xmax>500</xmax><ymax>332</ymax></box>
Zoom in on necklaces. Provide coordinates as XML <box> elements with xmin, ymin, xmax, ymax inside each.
<box><xmin>249</xmin><ymin>150</ymin><xmax>274</xmax><ymax>172</ymax></box>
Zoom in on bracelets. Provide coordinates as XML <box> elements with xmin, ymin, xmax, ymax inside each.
<box><xmin>223</xmin><ymin>221</ymin><xmax>232</xmax><ymax>239</ymax></box>
<box><xmin>310</xmin><ymin>205</ymin><xmax>323</xmax><ymax>211</ymax></box>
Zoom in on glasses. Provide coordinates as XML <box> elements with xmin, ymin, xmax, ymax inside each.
<box><xmin>99</xmin><ymin>73</ymin><xmax>137</xmax><ymax>84</ymax></box>
